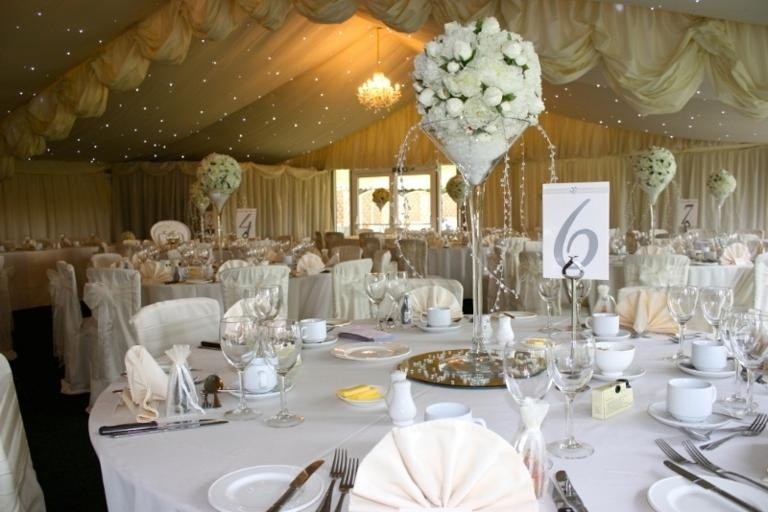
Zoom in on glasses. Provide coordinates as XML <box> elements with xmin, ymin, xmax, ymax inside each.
<box><xmin>355</xmin><ymin>23</ymin><xmax>402</xmax><ymax>114</ymax></box>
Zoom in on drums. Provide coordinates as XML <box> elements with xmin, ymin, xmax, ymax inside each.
<box><xmin>266</xmin><ymin>459</ymin><xmax>323</xmax><ymax>511</ymax></box>
<box><xmin>546</xmin><ymin>470</ymin><xmax>589</xmax><ymax>512</ymax></box>
<box><xmin>661</xmin><ymin>459</ymin><xmax>762</xmax><ymax>511</ymax></box>
<box><xmin>100</xmin><ymin>417</ymin><xmax>226</xmax><ymax>438</ymax></box>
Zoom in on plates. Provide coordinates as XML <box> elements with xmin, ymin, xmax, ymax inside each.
<box><xmin>226</xmin><ymin>378</ymin><xmax>294</xmax><ymax>398</ymax></box>
<box><xmin>595</xmin><ymin>330</ymin><xmax>632</xmax><ymax>341</ymax></box>
<box><xmin>208</xmin><ymin>465</ymin><xmax>322</xmax><ymax>512</ymax></box>
<box><xmin>330</xmin><ymin>342</ymin><xmax>411</xmax><ymax>362</ymax></box>
<box><xmin>648</xmin><ymin>401</ymin><xmax>731</xmax><ymax>432</ymax></box>
<box><xmin>676</xmin><ymin>359</ymin><xmax>736</xmax><ymax>380</ymax></box>
<box><xmin>301</xmin><ymin>333</ymin><xmax>339</xmax><ymax>348</ymax></box>
<box><xmin>339</xmin><ymin>385</ymin><xmax>387</xmax><ymax>409</ymax></box>
<box><xmin>592</xmin><ymin>367</ymin><xmax>647</xmax><ymax>383</ymax></box>
<box><xmin>494</xmin><ymin>309</ymin><xmax>538</xmax><ymax>320</ymax></box>
<box><xmin>416</xmin><ymin>323</ymin><xmax>464</xmax><ymax>333</ymax></box>
<box><xmin>647</xmin><ymin>475</ymin><xmax>768</xmax><ymax>512</ymax></box>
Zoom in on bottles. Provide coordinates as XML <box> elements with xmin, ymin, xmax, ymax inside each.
<box><xmin>386</xmin><ymin>372</ymin><xmax>418</xmax><ymax>426</ymax></box>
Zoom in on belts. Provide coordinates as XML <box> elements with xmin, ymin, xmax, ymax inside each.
<box><xmin>197</xmin><ymin>247</ymin><xmax>211</xmax><ymax>284</ymax></box>
<box><xmin>182</xmin><ymin>237</ymin><xmax>264</xmax><ymax>264</ymax></box>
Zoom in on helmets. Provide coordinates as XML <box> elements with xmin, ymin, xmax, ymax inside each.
<box><xmin>647</xmin><ymin>475</ymin><xmax>768</xmax><ymax>512</ymax></box>
<box><xmin>676</xmin><ymin>359</ymin><xmax>736</xmax><ymax>380</ymax></box>
<box><xmin>208</xmin><ymin>465</ymin><xmax>322</xmax><ymax>512</ymax></box>
<box><xmin>648</xmin><ymin>401</ymin><xmax>731</xmax><ymax>432</ymax></box>
<box><xmin>592</xmin><ymin>367</ymin><xmax>647</xmax><ymax>383</ymax></box>
<box><xmin>339</xmin><ymin>385</ymin><xmax>387</xmax><ymax>409</ymax></box>
<box><xmin>595</xmin><ymin>330</ymin><xmax>632</xmax><ymax>341</ymax></box>
<box><xmin>301</xmin><ymin>333</ymin><xmax>339</xmax><ymax>348</ymax></box>
<box><xmin>416</xmin><ymin>323</ymin><xmax>464</xmax><ymax>333</ymax></box>
<box><xmin>226</xmin><ymin>378</ymin><xmax>294</xmax><ymax>398</ymax></box>
<box><xmin>330</xmin><ymin>342</ymin><xmax>411</xmax><ymax>362</ymax></box>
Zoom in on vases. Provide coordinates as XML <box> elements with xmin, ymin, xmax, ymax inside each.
<box><xmin>187</xmin><ymin>175</ymin><xmax>210</xmax><ymax>210</ymax></box>
<box><xmin>411</xmin><ymin>13</ymin><xmax>547</xmax><ymax>188</ymax></box>
<box><xmin>712</xmin><ymin>192</ymin><xmax>728</xmax><ymax>235</ymax></box>
<box><xmin>439</xmin><ymin>174</ymin><xmax>468</xmax><ymax>203</ymax></box>
<box><xmin>452</xmin><ymin>199</ymin><xmax>465</xmax><ymax>243</ymax></box>
<box><xmin>635</xmin><ymin>177</ymin><xmax>665</xmax><ymax>245</ymax></box>
<box><xmin>209</xmin><ymin>189</ymin><xmax>231</xmax><ymax>257</ymax></box>
<box><xmin>634</xmin><ymin>144</ymin><xmax>677</xmax><ymax>199</ymax></box>
<box><xmin>397</xmin><ymin>115</ymin><xmax>547</xmax><ymax>390</ymax></box>
<box><xmin>707</xmin><ymin>167</ymin><xmax>738</xmax><ymax>202</ymax></box>
<box><xmin>195</xmin><ymin>151</ymin><xmax>242</xmax><ymax>209</ymax></box>
<box><xmin>372</xmin><ymin>185</ymin><xmax>391</xmax><ymax>207</ymax></box>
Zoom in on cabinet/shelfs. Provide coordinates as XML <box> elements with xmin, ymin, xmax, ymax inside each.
<box><xmin>386</xmin><ymin>372</ymin><xmax>418</xmax><ymax>426</ymax></box>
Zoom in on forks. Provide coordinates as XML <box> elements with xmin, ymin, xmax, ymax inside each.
<box><xmin>311</xmin><ymin>445</ymin><xmax>358</xmax><ymax>512</ymax></box>
<box><xmin>656</xmin><ymin>414</ymin><xmax>768</xmax><ymax>491</ymax></box>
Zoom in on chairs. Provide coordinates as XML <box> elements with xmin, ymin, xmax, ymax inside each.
<box><xmin>0</xmin><ymin>350</ymin><xmax>48</xmax><ymax>511</ymax></box>
<box><xmin>46</xmin><ymin>219</ymin><xmax>763</xmax><ymax>413</ymax></box>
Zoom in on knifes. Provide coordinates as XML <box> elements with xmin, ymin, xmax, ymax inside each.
<box><xmin>100</xmin><ymin>417</ymin><xmax>226</xmax><ymax>438</ymax></box>
<box><xmin>266</xmin><ymin>459</ymin><xmax>323</xmax><ymax>511</ymax></box>
<box><xmin>546</xmin><ymin>470</ymin><xmax>589</xmax><ymax>512</ymax></box>
<box><xmin>661</xmin><ymin>459</ymin><xmax>762</xmax><ymax>511</ymax></box>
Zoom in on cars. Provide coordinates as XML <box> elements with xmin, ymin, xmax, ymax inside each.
<box><xmin>586</xmin><ymin>314</ymin><xmax>619</xmax><ymax>337</ymax></box>
<box><xmin>693</xmin><ymin>340</ymin><xmax>728</xmax><ymax>372</ymax></box>
<box><xmin>244</xmin><ymin>358</ymin><xmax>276</xmax><ymax>394</ymax></box>
<box><xmin>421</xmin><ymin>307</ymin><xmax>452</xmax><ymax>328</ymax></box>
<box><xmin>299</xmin><ymin>318</ymin><xmax>326</xmax><ymax>343</ymax></box>
<box><xmin>668</xmin><ymin>378</ymin><xmax>717</xmax><ymax>422</ymax></box>
<box><xmin>595</xmin><ymin>342</ymin><xmax>636</xmax><ymax>377</ymax></box>
<box><xmin>425</xmin><ymin>403</ymin><xmax>486</xmax><ymax>428</ymax></box>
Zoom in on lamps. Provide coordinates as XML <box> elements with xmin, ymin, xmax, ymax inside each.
<box><xmin>355</xmin><ymin>23</ymin><xmax>402</xmax><ymax>114</ymax></box>
<box><xmin>0</xmin><ymin>350</ymin><xmax>48</xmax><ymax>511</ymax></box>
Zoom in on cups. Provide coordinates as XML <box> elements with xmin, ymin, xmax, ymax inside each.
<box><xmin>586</xmin><ymin>314</ymin><xmax>619</xmax><ymax>337</ymax></box>
<box><xmin>244</xmin><ymin>358</ymin><xmax>276</xmax><ymax>394</ymax></box>
<box><xmin>693</xmin><ymin>340</ymin><xmax>728</xmax><ymax>372</ymax></box>
<box><xmin>299</xmin><ymin>318</ymin><xmax>326</xmax><ymax>343</ymax></box>
<box><xmin>668</xmin><ymin>378</ymin><xmax>717</xmax><ymax>422</ymax></box>
<box><xmin>425</xmin><ymin>403</ymin><xmax>486</xmax><ymax>428</ymax></box>
<box><xmin>595</xmin><ymin>342</ymin><xmax>636</xmax><ymax>377</ymax></box>
<box><xmin>421</xmin><ymin>307</ymin><xmax>452</xmax><ymax>328</ymax></box>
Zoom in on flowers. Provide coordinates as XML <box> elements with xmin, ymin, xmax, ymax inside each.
<box><xmin>439</xmin><ymin>174</ymin><xmax>468</xmax><ymax>203</ymax></box>
<box><xmin>195</xmin><ymin>151</ymin><xmax>242</xmax><ymax>209</ymax></box>
<box><xmin>372</xmin><ymin>185</ymin><xmax>391</xmax><ymax>207</ymax></box>
<box><xmin>707</xmin><ymin>167</ymin><xmax>738</xmax><ymax>202</ymax></box>
<box><xmin>634</xmin><ymin>144</ymin><xmax>677</xmax><ymax>199</ymax></box>
<box><xmin>187</xmin><ymin>175</ymin><xmax>210</xmax><ymax>210</ymax></box>
<box><xmin>411</xmin><ymin>13</ymin><xmax>547</xmax><ymax>188</ymax></box>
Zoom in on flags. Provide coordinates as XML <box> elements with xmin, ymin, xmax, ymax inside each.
<box><xmin>712</xmin><ymin>192</ymin><xmax>728</xmax><ymax>235</ymax></box>
<box><xmin>209</xmin><ymin>189</ymin><xmax>231</xmax><ymax>257</ymax></box>
<box><xmin>452</xmin><ymin>199</ymin><xmax>465</xmax><ymax>243</ymax></box>
<box><xmin>397</xmin><ymin>115</ymin><xmax>547</xmax><ymax>390</ymax></box>
<box><xmin>635</xmin><ymin>177</ymin><xmax>665</xmax><ymax>245</ymax></box>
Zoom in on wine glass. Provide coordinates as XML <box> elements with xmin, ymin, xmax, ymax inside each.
<box><xmin>260</xmin><ymin>320</ymin><xmax>305</xmax><ymax>428</ymax></box>
<box><xmin>700</xmin><ymin>286</ymin><xmax>733</xmax><ymax>342</ymax></box>
<box><xmin>386</xmin><ymin>274</ymin><xmax>407</xmax><ymax>330</ymax></box>
<box><xmin>182</xmin><ymin>237</ymin><xmax>264</xmax><ymax>264</ymax></box>
<box><xmin>550</xmin><ymin>334</ymin><xmax>592</xmax><ymax>456</ymax></box>
<box><xmin>721</xmin><ymin>302</ymin><xmax>766</xmax><ymax>418</ymax></box>
<box><xmin>570</xmin><ymin>279</ymin><xmax>591</xmax><ymax>333</ymax></box>
<box><xmin>669</xmin><ymin>285</ymin><xmax>698</xmax><ymax>361</ymax></box>
<box><xmin>536</xmin><ymin>273</ymin><xmax>561</xmax><ymax>333</ymax></box>
<box><xmin>243</xmin><ymin>285</ymin><xmax>278</xmax><ymax>354</ymax></box>
<box><xmin>366</xmin><ymin>272</ymin><xmax>388</xmax><ymax>332</ymax></box>
<box><xmin>504</xmin><ymin>338</ymin><xmax>553</xmax><ymax>429</ymax></box>
<box><xmin>221</xmin><ymin>316</ymin><xmax>263</xmax><ymax>421</ymax></box>
<box><xmin>197</xmin><ymin>247</ymin><xmax>211</xmax><ymax>284</ymax></box>
